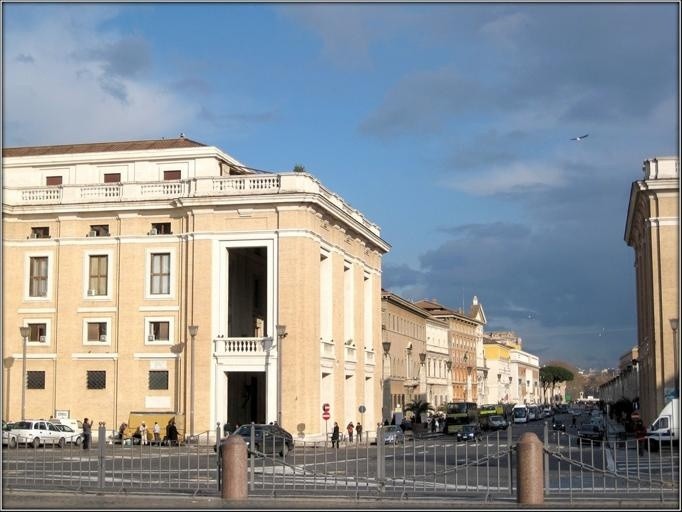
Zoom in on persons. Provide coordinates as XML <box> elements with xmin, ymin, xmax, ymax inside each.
<box><xmin>122</xmin><ymin>426</ymin><xmax>129</xmax><ymax>444</ymax></box>
<box><xmin>132</xmin><ymin>420</ymin><xmax>182</xmax><ymax>445</ymax></box>
<box><xmin>224</xmin><ymin>422</ymin><xmax>231</xmax><ymax>437</ymax></box>
<box><xmin>356</xmin><ymin>422</ymin><xmax>362</xmax><ymax>442</ymax></box>
<box><xmin>83</xmin><ymin>418</ymin><xmax>93</xmax><ymax>449</ymax></box>
<box><xmin>570</xmin><ymin>416</ymin><xmax>576</xmax><ymax>428</ymax></box>
<box><xmin>346</xmin><ymin>422</ymin><xmax>354</xmax><ymax>443</ymax></box>
<box><xmin>331</xmin><ymin>422</ymin><xmax>340</xmax><ymax>448</ymax></box>
<box><xmin>430</xmin><ymin>418</ymin><xmax>436</xmax><ymax>433</ymax></box>
<box><xmin>634</xmin><ymin>420</ymin><xmax>646</xmax><ymax>456</ymax></box>
<box><xmin>232</xmin><ymin>424</ymin><xmax>243</xmax><ymax>435</ymax></box>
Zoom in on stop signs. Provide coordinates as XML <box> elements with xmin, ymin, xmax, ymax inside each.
<box><xmin>322</xmin><ymin>403</ymin><xmax>330</xmax><ymax>412</ymax></box>
<box><xmin>631</xmin><ymin>411</ymin><xmax>641</xmax><ymax>423</ymax></box>
<box><xmin>323</xmin><ymin>412</ymin><xmax>330</xmax><ymax>421</ymax></box>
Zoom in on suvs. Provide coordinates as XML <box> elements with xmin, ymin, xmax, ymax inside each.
<box><xmin>456</xmin><ymin>425</ymin><xmax>482</xmax><ymax>441</ymax></box>
<box><xmin>577</xmin><ymin>423</ymin><xmax>602</xmax><ymax>447</ymax></box>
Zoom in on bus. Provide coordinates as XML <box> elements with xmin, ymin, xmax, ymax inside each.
<box><xmin>447</xmin><ymin>400</ymin><xmax>568</xmax><ymax>434</ymax></box>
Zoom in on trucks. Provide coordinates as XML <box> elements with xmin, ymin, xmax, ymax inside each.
<box><xmin>644</xmin><ymin>397</ymin><xmax>680</xmax><ymax>452</ymax></box>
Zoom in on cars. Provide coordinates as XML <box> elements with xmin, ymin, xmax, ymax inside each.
<box><xmin>54</xmin><ymin>422</ymin><xmax>85</xmax><ymax>446</ymax></box>
<box><xmin>12</xmin><ymin>418</ymin><xmax>65</xmax><ymax>448</ymax></box>
<box><xmin>374</xmin><ymin>425</ymin><xmax>405</xmax><ymax>445</ymax></box>
<box><xmin>2</xmin><ymin>419</ymin><xmax>18</xmax><ymax>449</ymax></box>
<box><xmin>213</xmin><ymin>422</ymin><xmax>295</xmax><ymax>458</ymax></box>
<box><xmin>568</xmin><ymin>401</ymin><xmax>605</xmax><ymax>424</ymax></box>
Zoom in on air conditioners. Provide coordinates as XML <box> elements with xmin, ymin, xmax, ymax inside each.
<box><xmin>88</xmin><ymin>231</ymin><xmax>96</xmax><ymax>237</ymax></box>
<box><xmin>150</xmin><ymin>229</ymin><xmax>157</xmax><ymax>235</ymax></box>
<box><xmin>148</xmin><ymin>335</ymin><xmax>155</xmax><ymax>341</ymax></box>
<box><xmin>31</xmin><ymin>233</ymin><xmax>38</xmax><ymax>238</ymax></box>
<box><xmin>100</xmin><ymin>334</ymin><xmax>107</xmax><ymax>342</ymax></box>
<box><xmin>40</xmin><ymin>336</ymin><xmax>46</xmax><ymax>342</ymax></box>
<box><xmin>87</xmin><ymin>289</ymin><xmax>96</xmax><ymax>296</ymax></box>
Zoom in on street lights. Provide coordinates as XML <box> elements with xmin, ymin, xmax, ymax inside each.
<box><xmin>667</xmin><ymin>319</ymin><xmax>679</xmax><ymax>394</ymax></box>
